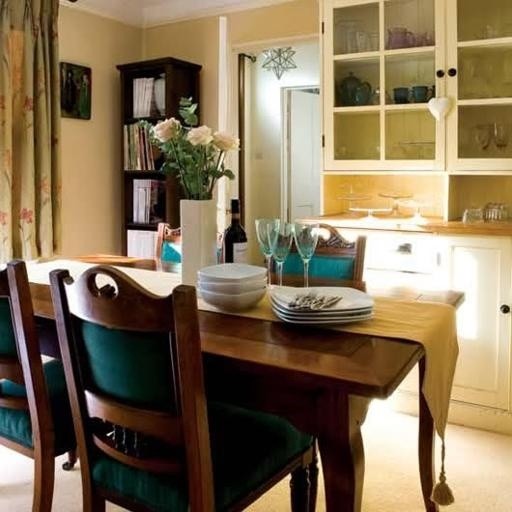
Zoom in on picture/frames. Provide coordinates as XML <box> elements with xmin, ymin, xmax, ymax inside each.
<box><xmin>60</xmin><ymin>62</ymin><xmax>92</xmax><ymax>120</ymax></box>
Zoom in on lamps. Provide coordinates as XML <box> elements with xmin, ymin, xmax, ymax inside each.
<box><xmin>262</xmin><ymin>46</ymin><xmax>298</xmax><ymax>81</ymax></box>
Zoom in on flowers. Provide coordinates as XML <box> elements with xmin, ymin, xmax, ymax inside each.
<box><xmin>139</xmin><ymin>96</ymin><xmax>241</xmax><ymax>200</ymax></box>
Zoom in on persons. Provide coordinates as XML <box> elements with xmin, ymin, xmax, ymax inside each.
<box><xmin>65</xmin><ymin>71</ymin><xmax>89</xmax><ymax>117</ymax></box>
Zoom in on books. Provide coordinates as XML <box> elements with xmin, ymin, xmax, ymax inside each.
<box><xmin>123</xmin><ymin>77</ymin><xmax>167</xmax><ymax>225</ymax></box>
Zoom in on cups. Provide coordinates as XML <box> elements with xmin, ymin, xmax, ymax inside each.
<box><xmin>392</xmin><ymin>86</ymin><xmax>433</xmax><ymax>103</ymax></box>
<box><xmin>353</xmin><ymin>31</ymin><xmax>379</xmax><ymax>53</ymax></box>
<box><xmin>386</xmin><ymin>28</ymin><xmax>433</xmax><ymax>49</ymax></box>
<box><xmin>484</xmin><ymin>201</ymin><xmax>511</xmax><ymax>226</ymax></box>
<box><xmin>334</xmin><ymin>145</ymin><xmax>352</xmax><ymax>159</ymax></box>
<box><xmin>462</xmin><ymin>207</ymin><xmax>484</xmax><ymax>229</ymax></box>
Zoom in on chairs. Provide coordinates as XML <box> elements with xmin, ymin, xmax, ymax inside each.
<box><xmin>49</xmin><ymin>267</ymin><xmax>319</xmax><ymax>512</ymax></box>
<box><xmin>154</xmin><ymin>223</ymin><xmax>224</xmax><ymax>272</ymax></box>
<box><xmin>262</xmin><ymin>223</ymin><xmax>366</xmax><ymax>289</ymax></box>
<box><xmin>0</xmin><ymin>260</ymin><xmax>77</xmax><ymax>511</ymax></box>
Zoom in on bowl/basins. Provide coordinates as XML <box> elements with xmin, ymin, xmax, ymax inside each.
<box><xmin>198</xmin><ymin>262</ymin><xmax>267</xmax><ymax>313</ymax></box>
<box><xmin>399</xmin><ymin>142</ymin><xmax>435</xmax><ymax>159</ymax></box>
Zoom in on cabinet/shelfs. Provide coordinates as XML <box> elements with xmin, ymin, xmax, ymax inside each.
<box><xmin>294</xmin><ymin>0</ymin><xmax>512</xmax><ymax>435</ymax></box>
<box><xmin>116</xmin><ymin>57</ymin><xmax>203</xmax><ymax>258</ymax></box>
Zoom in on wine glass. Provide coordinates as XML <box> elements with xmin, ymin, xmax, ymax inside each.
<box><xmin>474</xmin><ymin>121</ymin><xmax>510</xmax><ymax>157</ymax></box>
<box><xmin>255</xmin><ymin>217</ymin><xmax>321</xmax><ymax>286</ymax></box>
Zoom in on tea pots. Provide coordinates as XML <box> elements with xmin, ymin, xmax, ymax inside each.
<box><xmin>335</xmin><ymin>72</ymin><xmax>372</xmax><ymax>106</ymax></box>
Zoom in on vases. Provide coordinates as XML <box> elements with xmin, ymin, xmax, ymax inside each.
<box><xmin>178</xmin><ymin>199</ymin><xmax>218</xmax><ymax>286</ymax></box>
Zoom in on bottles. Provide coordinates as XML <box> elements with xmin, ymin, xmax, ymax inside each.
<box><xmin>222</xmin><ymin>199</ymin><xmax>248</xmax><ymax>263</ymax></box>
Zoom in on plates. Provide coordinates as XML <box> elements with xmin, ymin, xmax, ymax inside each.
<box><xmin>267</xmin><ymin>286</ymin><xmax>376</xmax><ymax>324</ymax></box>
